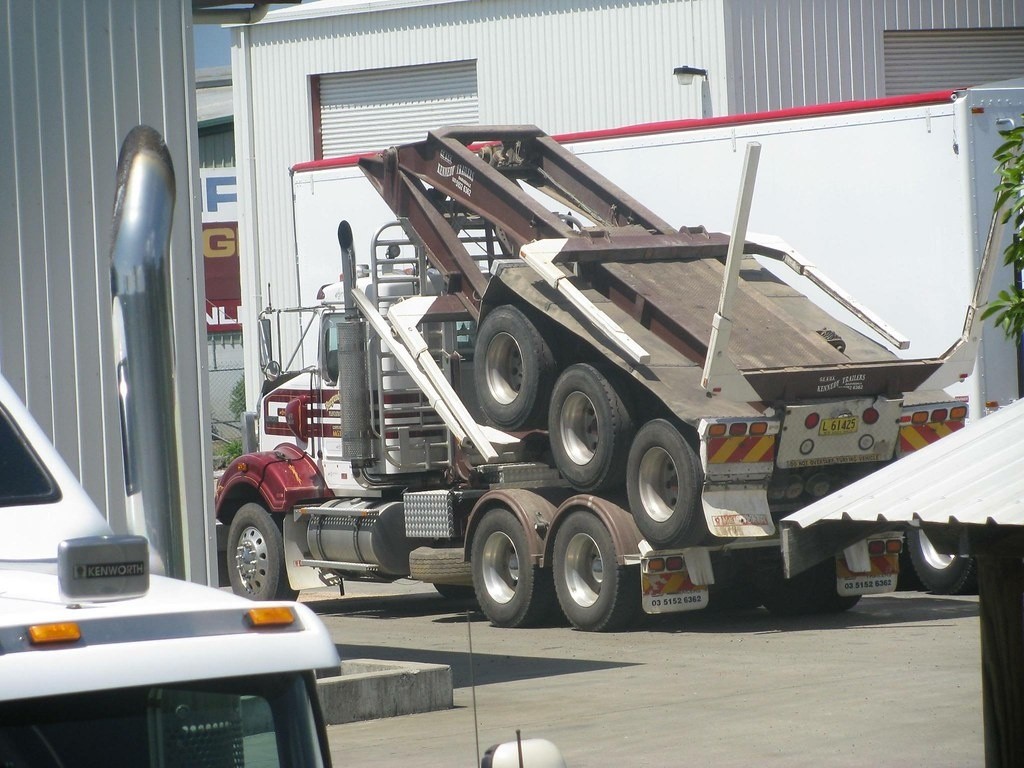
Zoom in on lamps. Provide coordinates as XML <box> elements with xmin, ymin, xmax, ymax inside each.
<box><xmin>675</xmin><ymin>64</ymin><xmax>708</xmax><ymax>85</ymax></box>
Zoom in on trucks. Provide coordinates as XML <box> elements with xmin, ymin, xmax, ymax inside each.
<box><xmin>255</xmin><ymin>73</ymin><xmax>1023</xmax><ymax>595</ymax></box>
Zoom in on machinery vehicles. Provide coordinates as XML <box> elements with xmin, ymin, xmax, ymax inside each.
<box><xmin>1</xmin><ymin>124</ymin><xmax>342</xmax><ymax>768</ymax></box>
<box><xmin>214</xmin><ymin>123</ymin><xmax>947</xmax><ymax>633</ymax></box>
